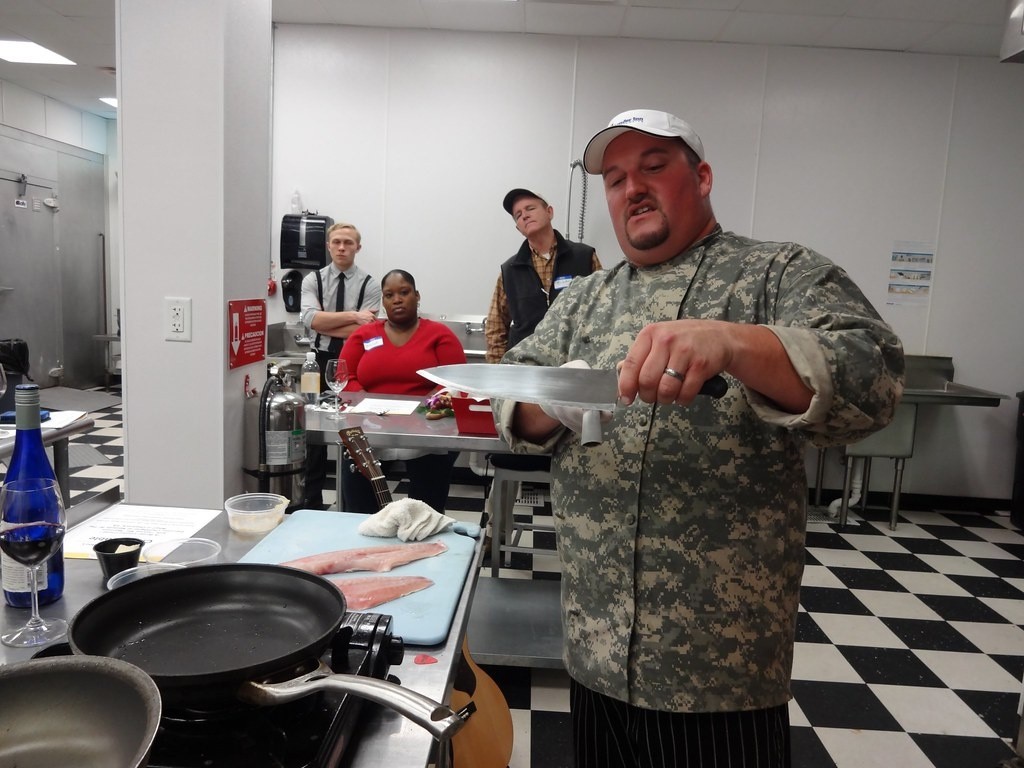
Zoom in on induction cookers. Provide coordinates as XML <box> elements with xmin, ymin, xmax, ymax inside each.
<box><xmin>24</xmin><ymin>599</ymin><xmax>398</xmax><ymax>768</ymax></box>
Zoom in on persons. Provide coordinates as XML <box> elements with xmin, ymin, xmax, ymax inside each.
<box><xmin>485</xmin><ymin>189</ymin><xmax>603</xmax><ymax>364</ymax></box>
<box><xmin>490</xmin><ymin>108</ymin><xmax>904</xmax><ymax>768</ymax></box>
<box><xmin>300</xmin><ymin>223</ymin><xmax>382</xmax><ymax>508</ymax></box>
<box><xmin>335</xmin><ymin>270</ymin><xmax>467</xmax><ymax>513</ymax></box>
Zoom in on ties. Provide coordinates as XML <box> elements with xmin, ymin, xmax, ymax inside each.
<box><xmin>327</xmin><ymin>272</ymin><xmax>343</xmax><ymax>359</ymax></box>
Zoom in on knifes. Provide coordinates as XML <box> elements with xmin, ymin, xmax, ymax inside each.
<box><xmin>416</xmin><ymin>356</ymin><xmax>729</xmax><ymax>409</ymax></box>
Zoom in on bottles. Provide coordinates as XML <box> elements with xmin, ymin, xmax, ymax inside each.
<box><xmin>0</xmin><ymin>382</ymin><xmax>65</xmax><ymax>609</ymax></box>
<box><xmin>301</xmin><ymin>352</ymin><xmax>320</xmax><ymax>409</ymax></box>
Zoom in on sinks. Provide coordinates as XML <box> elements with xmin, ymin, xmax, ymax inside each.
<box><xmin>901</xmin><ymin>373</ymin><xmax>1011</xmax><ymax>408</ymax></box>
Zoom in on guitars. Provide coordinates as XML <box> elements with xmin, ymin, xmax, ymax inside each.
<box><xmin>337</xmin><ymin>426</ymin><xmax>515</xmax><ymax>767</ymax></box>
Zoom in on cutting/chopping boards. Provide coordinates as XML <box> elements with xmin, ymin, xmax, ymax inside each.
<box><xmin>225</xmin><ymin>511</ymin><xmax>481</xmax><ymax>648</ymax></box>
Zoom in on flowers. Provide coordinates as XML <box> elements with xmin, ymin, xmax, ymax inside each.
<box><xmin>417</xmin><ymin>393</ymin><xmax>444</xmax><ymax>411</ymax></box>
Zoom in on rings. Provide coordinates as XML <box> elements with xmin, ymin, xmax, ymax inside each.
<box><xmin>664</xmin><ymin>368</ymin><xmax>685</xmax><ymax>382</ymax></box>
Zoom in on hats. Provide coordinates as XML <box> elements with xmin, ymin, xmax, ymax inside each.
<box><xmin>583</xmin><ymin>109</ymin><xmax>706</xmax><ymax>174</ymax></box>
<box><xmin>502</xmin><ymin>188</ymin><xmax>549</xmax><ymax>214</ymax></box>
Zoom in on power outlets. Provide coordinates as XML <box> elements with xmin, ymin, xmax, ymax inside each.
<box><xmin>164</xmin><ymin>297</ymin><xmax>193</xmax><ymax>342</ymax></box>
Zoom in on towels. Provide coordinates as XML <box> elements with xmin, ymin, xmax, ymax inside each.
<box><xmin>356</xmin><ymin>498</ymin><xmax>457</xmax><ymax>542</ymax></box>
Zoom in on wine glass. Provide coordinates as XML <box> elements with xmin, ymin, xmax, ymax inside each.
<box><xmin>0</xmin><ymin>478</ymin><xmax>69</xmax><ymax>648</ymax></box>
<box><xmin>324</xmin><ymin>359</ymin><xmax>346</xmax><ymax>420</ymax></box>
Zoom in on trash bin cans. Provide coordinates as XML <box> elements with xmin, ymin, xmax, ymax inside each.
<box><xmin>0</xmin><ymin>338</ymin><xmax>29</xmax><ymax>410</ymax></box>
<box><xmin>1009</xmin><ymin>390</ymin><xmax>1024</xmax><ymax>529</ymax></box>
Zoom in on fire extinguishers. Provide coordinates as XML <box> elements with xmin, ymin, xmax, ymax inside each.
<box><xmin>258</xmin><ymin>360</ymin><xmax>307</xmax><ymax>510</ymax></box>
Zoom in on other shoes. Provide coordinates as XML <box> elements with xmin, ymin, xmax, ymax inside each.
<box><xmin>302</xmin><ymin>496</ymin><xmax>323</xmax><ymax>510</ymax></box>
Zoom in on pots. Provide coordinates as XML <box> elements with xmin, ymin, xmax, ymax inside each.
<box><xmin>62</xmin><ymin>561</ymin><xmax>464</xmax><ymax>743</ymax></box>
<box><xmin>0</xmin><ymin>656</ymin><xmax>164</xmax><ymax>768</ymax></box>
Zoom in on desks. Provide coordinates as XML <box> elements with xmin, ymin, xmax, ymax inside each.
<box><xmin>0</xmin><ymin>417</ymin><xmax>95</xmax><ymax>512</ymax></box>
<box><xmin>300</xmin><ymin>390</ymin><xmax>555</xmax><ymax>512</ymax></box>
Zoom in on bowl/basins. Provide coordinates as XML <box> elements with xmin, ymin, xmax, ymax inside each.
<box><xmin>107</xmin><ymin>564</ymin><xmax>187</xmax><ymax>593</ymax></box>
<box><xmin>223</xmin><ymin>493</ymin><xmax>289</xmax><ymax>533</ymax></box>
<box><xmin>91</xmin><ymin>538</ymin><xmax>144</xmax><ymax>578</ymax></box>
<box><xmin>142</xmin><ymin>537</ymin><xmax>221</xmax><ymax>570</ymax></box>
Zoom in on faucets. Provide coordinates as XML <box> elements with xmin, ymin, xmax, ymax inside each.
<box><xmin>464</xmin><ymin>317</ymin><xmax>488</xmax><ymax>335</ymax></box>
